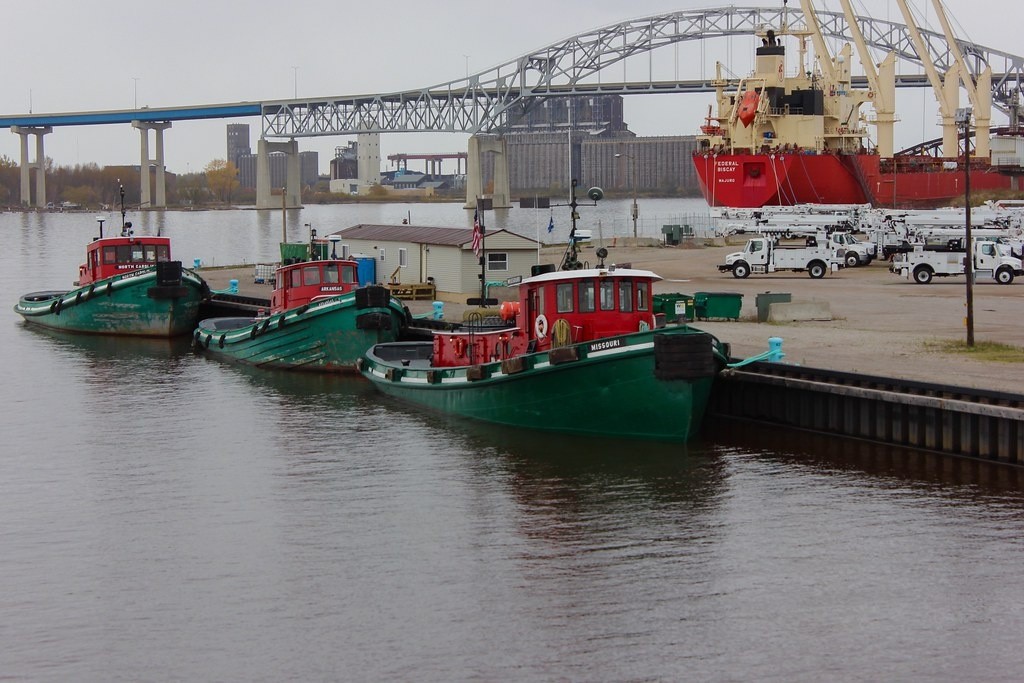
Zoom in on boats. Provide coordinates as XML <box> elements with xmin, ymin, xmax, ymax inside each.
<box><xmin>353</xmin><ymin>178</ymin><xmax>735</xmax><ymax>440</ymax></box>
<box><xmin>14</xmin><ymin>187</ymin><xmax>211</xmax><ymax>337</ymax></box>
<box><xmin>196</xmin><ymin>227</ymin><xmax>410</xmax><ymax>374</ymax></box>
<box><xmin>691</xmin><ymin>0</ymin><xmax>1024</xmax><ymax>210</ymax></box>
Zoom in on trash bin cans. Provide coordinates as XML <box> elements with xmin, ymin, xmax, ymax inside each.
<box><xmin>693</xmin><ymin>292</ymin><xmax>743</xmax><ymax>322</ymax></box>
<box><xmin>311</xmin><ymin>239</ymin><xmax>328</xmax><ymax>261</ymax></box>
<box><xmin>280</xmin><ymin>241</ymin><xmax>309</xmax><ymax>266</ymax></box>
<box><xmin>652</xmin><ymin>292</ymin><xmax>694</xmax><ymax>321</ymax></box>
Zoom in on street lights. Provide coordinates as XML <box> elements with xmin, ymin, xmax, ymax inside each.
<box><xmin>290</xmin><ymin>64</ymin><xmax>299</xmax><ymax>97</ymax></box>
<box><xmin>463</xmin><ymin>53</ymin><xmax>470</xmax><ymax>86</ymax></box>
<box><xmin>130</xmin><ymin>77</ymin><xmax>140</xmax><ymax>107</ymax></box>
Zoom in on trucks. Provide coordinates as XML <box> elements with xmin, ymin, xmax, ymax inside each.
<box><xmin>712</xmin><ymin>200</ymin><xmax>1024</xmax><ymax>285</ymax></box>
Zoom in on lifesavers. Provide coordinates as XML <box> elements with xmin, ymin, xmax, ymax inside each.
<box><xmin>76</xmin><ymin>291</ymin><xmax>82</xmax><ymax>305</ymax></box>
<box><xmin>194</xmin><ymin>332</ymin><xmax>200</xmax><ymax>346</ymax></box>
<box><xmin>219</xmin><ymin>334</ymin><xmax>226</xmax><ymax>348</ymax></box>
<box><xmin>50</xmin><ymin>299</ymin><xmax>64</xmax><ymax>315</ymax></box>
<box><xmin>454</xmin><ymin>337</ymin><xmax>463</xmax><ymax>357</ymax></box>
<box><xmin>296</xmin><ymin>306</ymin><xmax>309</xmax><ymax>316</ymax></box>
<box><xmin>261</xmin><ymin>320</ymin><xmax>270</xmax><ymax>334</ymax></box>
<box><xmin>107</xmin><ymin>282</ymin><xmax>112</xmax><ymax>297</ymax></box>
<box><xmin>86</xmin><ymin>285</ymin><xmax>95</xmax><ymax>301</ymax></box>
<box><xmin>279</xmin><ymin>316</ymin><xmax>285</xmax><ymax>328</ymax></box>
<box><xmin>535</xmin><ymin>314</ymin><xmax>548</xmax><ymax>339</ymax></box>
<box><xmin>205</xmin><ymin>335</ymin><xmax>212</xmax><ymax>348</ymax></box>
<box><xmin>252</xmin><ymin>326</ymin><xmax>258</xmax><ymax>340</ymax></box>
